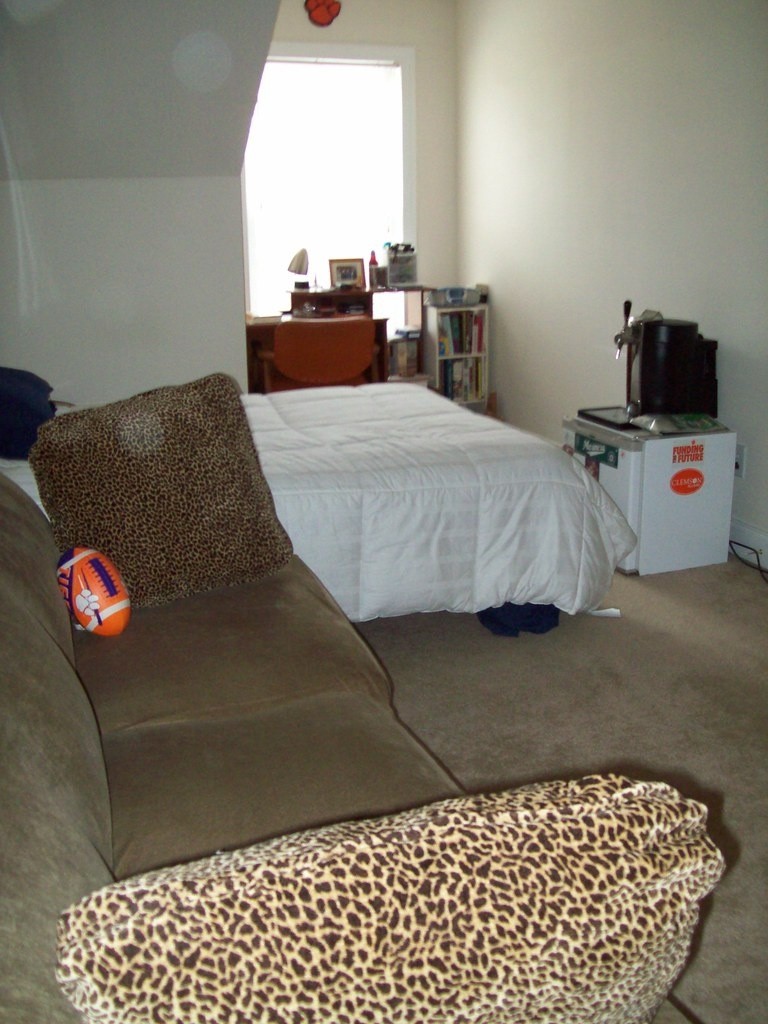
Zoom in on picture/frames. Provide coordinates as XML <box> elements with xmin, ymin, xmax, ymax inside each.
<box><xmin>329</xmin><ymin>258</ymin><xmax>365</xmax><ymax>287</ymax></box>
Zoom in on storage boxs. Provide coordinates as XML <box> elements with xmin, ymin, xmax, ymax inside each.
<box><xmin>387</xmin><ymin>255</ymin><xmax>417</xmax><ymax>286</ymax></box>
<box><xmin>388</xmin><ymin>338</ymin><xmax>417</xmax><ymax>379</ymax></box>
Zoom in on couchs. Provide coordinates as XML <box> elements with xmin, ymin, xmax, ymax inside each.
<box><xmin>0</xmin><ymin>471</ymin><xmax>691</xmax><ymax>1024</ymax></box>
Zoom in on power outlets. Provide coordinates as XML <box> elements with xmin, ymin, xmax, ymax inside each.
<box><xmin>735</xmin><ymin>443</ymin><xmax>746</xmax><ymax>479</ymax></box>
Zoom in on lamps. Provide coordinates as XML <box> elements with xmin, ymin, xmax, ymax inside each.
<box><xmin>289</xmin><ymin>249</ymin><xmax>309</xmax><ymax>289</ymax></box>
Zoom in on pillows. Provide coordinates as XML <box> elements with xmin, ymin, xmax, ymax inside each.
<box><xmin>0</xmin><ymin>367</ymin><xmax>57</xmax><ymax>460</ymax></box>
<box><xmin>51</xmin><ymin>771</ymin><xmax>732</xmax><ymax>1024</ymax></box>
<box><xmin>29</xmin><ymin>374</ymin><xmax>293</xmax><ymax>608</ymax></box>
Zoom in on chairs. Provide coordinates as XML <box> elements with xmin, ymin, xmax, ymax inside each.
<box><xmin>274</xmin><ymin>317</ymin><xmax>377</xmax><ymax>391</ymax></box>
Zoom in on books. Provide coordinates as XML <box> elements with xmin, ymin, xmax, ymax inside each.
<box><xmin>439</xmin><ymin>310</ymin><xmax>483</xmax><ymax>355</ymax></box>
<box><xmin>440</xmin><ymin>357</ymin><xmax>483</xmax><ymax>401</ymax></box>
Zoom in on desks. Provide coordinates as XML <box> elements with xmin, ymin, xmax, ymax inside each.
<box><xmin>247</xmin><ymin>314</ymin><xmax>388</xmax><ymax>394</ymax></box>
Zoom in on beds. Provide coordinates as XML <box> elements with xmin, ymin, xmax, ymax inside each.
<box><xmin>0</xmin><ymin>383</ymin><xmax>636</xmax><ymax>635</ymax></box>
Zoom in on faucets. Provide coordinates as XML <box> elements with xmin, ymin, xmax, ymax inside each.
<box><xmin>627</xmin><ymin>400</ymin><xmax>639</xmax><ymax>421</ymax></box>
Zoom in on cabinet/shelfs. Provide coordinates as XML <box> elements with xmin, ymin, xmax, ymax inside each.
<box><xmin>561</xmin><ymin>415</ymin><xmax>739</xmax><ymax>576</ymax></box>
<box><xmin>291</xmin><ymin>285</ymin><xmax>423</xmax><ymax>384</ymax></box>
<box><xmin>423</xmin><ymin>304</ymin><xmax>490</xmax><ymax>412</ymax></box>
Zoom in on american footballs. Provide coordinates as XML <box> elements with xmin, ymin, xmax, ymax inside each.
<box><xmin>54</xmin><ymin>544</ymin><xmax>133</xmax><ymax>638</ymax></box>
